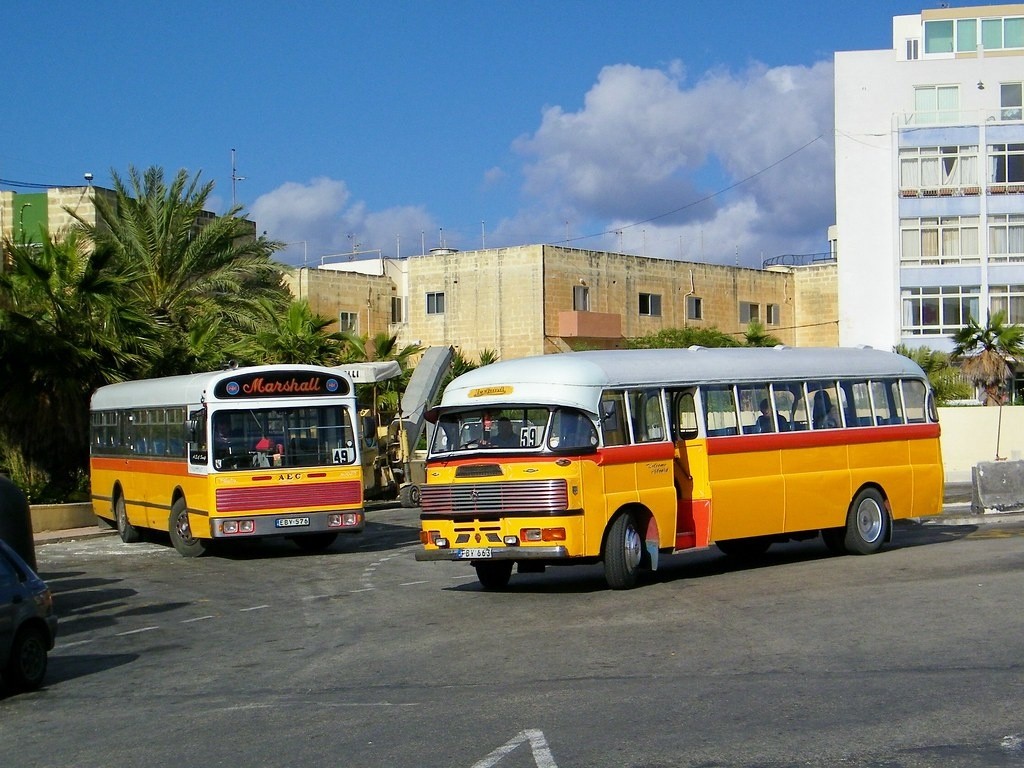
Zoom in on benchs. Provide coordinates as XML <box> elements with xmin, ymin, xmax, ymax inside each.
<box><xmin>96</xmin><ymin>436</ymin><xmax>186</xmax><ymax>456</ymax></box>
<box><xmin>711</xmin><ymin>415</ymin><xmax>909</xmax><ymax>435</ymax></box>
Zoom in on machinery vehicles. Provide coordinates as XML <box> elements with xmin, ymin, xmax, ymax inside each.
<box><xmin>292</xmin><ymin>345</ymin><xmax>455</xmax><ymax>509</ymax></box>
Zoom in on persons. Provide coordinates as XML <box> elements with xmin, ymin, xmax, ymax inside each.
<box><xmin>757</xmin><ymin>398</ymin><xmax>791</xmax><ymax>433</ymax></box>
<box><xmin>486</xmin><ymin>417</ymin><xmax>520</xmax><ymax>447</ymax></box>
<box><xmin>813</xmin><ymin>391</ymin><xmax>842</xmax><ymax>430</ymax></box>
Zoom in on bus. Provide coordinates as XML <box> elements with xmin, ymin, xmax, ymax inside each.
<box><xmin>414</xmin><ymin>345</ymin><xmax>944</xmax><ymax>591</ymax></box>
<box><xmin>88</xmin><ymin>363</ymin><xmax>375</xmax><ymax>558</ymax></box>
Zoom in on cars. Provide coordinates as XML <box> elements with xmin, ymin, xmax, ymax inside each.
<box><xmin>0</xmin><ymin>540</ymin><xmax>57</xmax><ymax>692</ymax></box>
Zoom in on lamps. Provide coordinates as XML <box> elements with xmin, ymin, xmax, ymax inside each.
<box><xmin>84</xmin><ymin>172</ymin><xmax>93</xmax><ymax>186</ymax></box>
